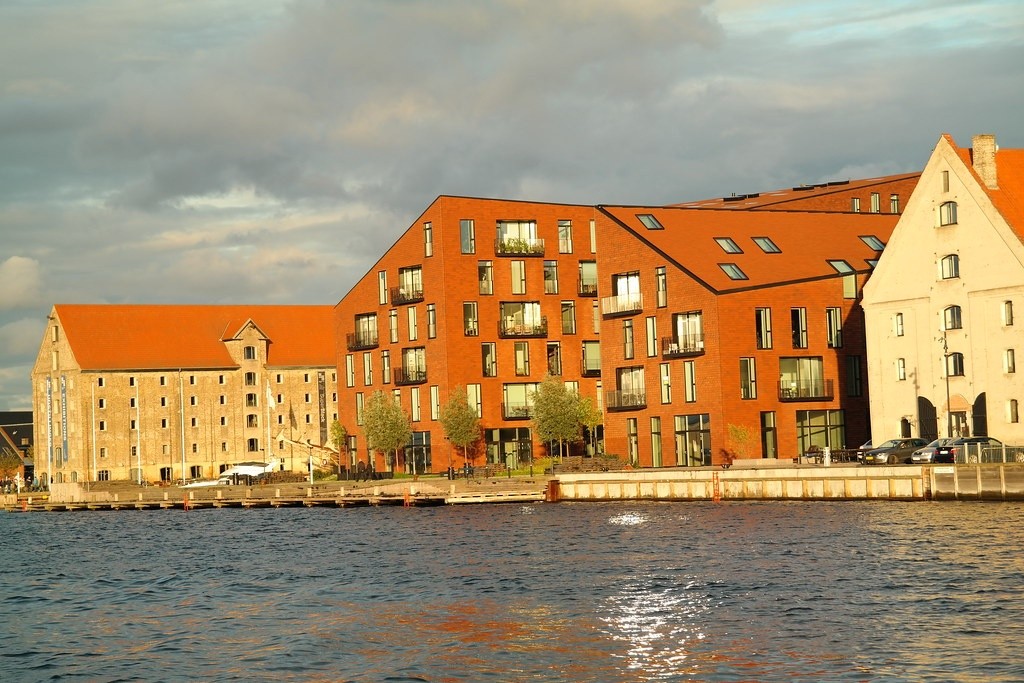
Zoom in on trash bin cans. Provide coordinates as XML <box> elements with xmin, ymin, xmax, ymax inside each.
<box><xmin>464</xmin><ymin>462</ymin><xmax>471</xmax><ymax>478</ymax></box>
<box><xmin>233</xmin><ymin>473</ymin><xmax>239</xmax><ymax>485</ymax></box>
<box><xmin>447</xmin><ymin>467</ymin><xmax>455</xmax><ymax>480</ymax></box>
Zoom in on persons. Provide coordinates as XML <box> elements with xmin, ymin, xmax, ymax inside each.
<box><xmin>1</xmin><ymin>475</ymin><xmax>43</xmax><ymax>492</ymax></box>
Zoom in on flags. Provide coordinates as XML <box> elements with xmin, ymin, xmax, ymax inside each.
<box><xmin>265</xmin><ymin>380</ymin><xmax>277</xmax><ymax>411</ymax></box>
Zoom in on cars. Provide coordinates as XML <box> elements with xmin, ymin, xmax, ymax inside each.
<box><xmin>858</xmin><ymin>438</ymin><xmax>873</xmax><ymax>464</ymax></box>
<box><xmin>863</xmin><ymin>437</ymin><xmax>931</xmax><ymax>465</ymax></box>
<box><xmin>910</xmin><ymin>436</ymin><xmax>955</xmax><ymax>463</ymax></box>
<box><xmin>934</xmin><ymin>435</ymin><xmax>1024</xmax><ymax>463</ymax></box>
